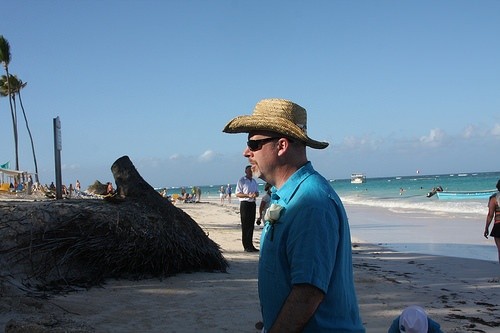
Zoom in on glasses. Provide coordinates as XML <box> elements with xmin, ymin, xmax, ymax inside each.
<box><xmin>248</xmin><ymin>138</ymin><xmax>276</xmax><ymax>151</ymax></box>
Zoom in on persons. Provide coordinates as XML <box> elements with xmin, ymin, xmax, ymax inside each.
<box><xmin>218</xmin><ymin>184</ymin><xmax>232</xmax><ymax>206</ymax></box>
<box><xmin>106</xmin><ymin>182</ymin><xmax>115</xmax><ymax>196</ymax></box>
<box><xmin>62</xmin><ymin>180</ymin><xmax>81</xmax><ymax>195</ymax></box>
<box><xmin>49</xmin><ymin>182</ymin><xmax>55</xmax><ymax>190</ymax></box>
<box><xmin>159</xmin><ymin>186</ymin><xmax>201</xmax><ymax>203</ymax></box>
<box><xmin>483</xmin><ymin>180</ymin><xmax>500</xmax><ymax>265</ymax></box>
<box><xmin>28</xmin><ymin>174</ymin><xmax>33</xmax><ymax>192</ymax></box>
<box><xmin>235</xmin><ymin>99</ymin><xmax>366</xmax><ymax>333</ymax></box>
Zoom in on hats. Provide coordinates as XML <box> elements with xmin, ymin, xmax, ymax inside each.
<box><xmin>264</xmin><ymin>182</ymin><xmax>272</xmax><ymax>191</ymax></box>
<box><xmin>223</xmin><ymin>99</ymin><xmax>329</xmax><ymax>149</ymax></box>
<box><xmin>399</xmin><ymin>305</ymin><xmax>428</xmax><ymax>333</ymax></box>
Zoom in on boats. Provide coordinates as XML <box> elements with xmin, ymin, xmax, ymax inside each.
<box><xmin>425</xmin><ymin>186</ymin><xmax>498</xmax><ymax>205</ymax></box>
<box><xmin>350</xmin><ymin>173</ymin><xmax>366</xmax><ymax>185</ymax></box>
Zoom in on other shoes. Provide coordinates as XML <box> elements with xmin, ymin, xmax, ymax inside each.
<box><xmin>246</xmin><ymin>247</ymin><xmax>259</xmax><ymax>252</ymax></box>
